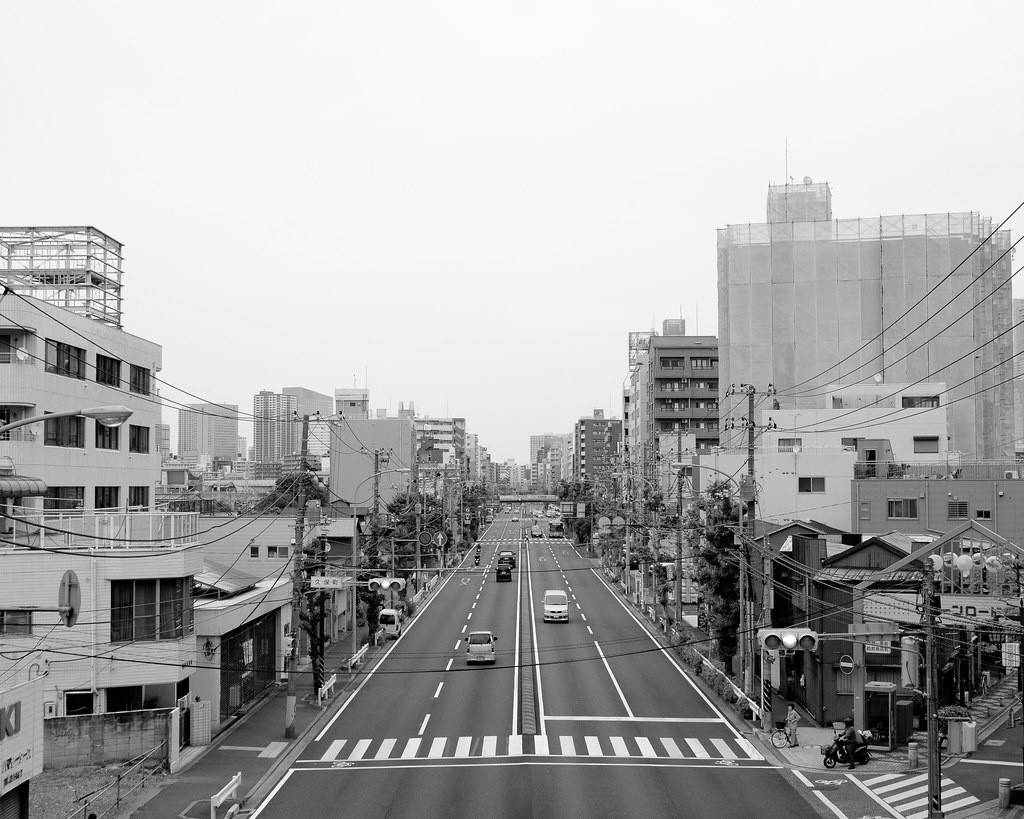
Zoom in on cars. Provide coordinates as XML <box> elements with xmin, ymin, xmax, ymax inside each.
<box><xmin>512</xmin><ymin>517</ymin><xmax>520</xmax><ymax>523</ymax></box>
<box><xmin>486</xmin><ymin>517</ymin><xmax>493</xmax><ymax>523</ymax></box>
<box><xmin>532</xmin><ymin>528</ymin><xmax>543</xmax><ymax>538</ymax></box>
<box><xmin>503</xmin><ymin>502</ymin><xmax>519</xmax><ymax>514</ymax></box>
<box><xmin>533</xmin><ymin>510</ymin><xmax>562</xmax><ymax>519</ymax></box>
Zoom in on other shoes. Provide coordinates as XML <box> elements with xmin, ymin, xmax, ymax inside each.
<box><xmin>795</xmin><ymin>744</ymin><xmax>799</xmax><ymax>747</ymax></box>
<box><xmin>840</xmin><ymin>753</ymin><xmax>846</xmax><ymax>758</ymax></box>
<box><xmin>788</xmin><ymin>745</ymin><xmax>795</xmax><ymax>748</ymax></box>
<box><xmin>848</xmin><ymin>764</ymin><xmax>856</xmax><ymax>769</ymax></box>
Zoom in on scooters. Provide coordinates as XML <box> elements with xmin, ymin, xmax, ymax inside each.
<box><xmin>474</xmin><ymin>554</ymin><xmax>480</xmax><ymax>567</ymax></box>
<box><xmin>820</xmin><ymin>729</ymin><xmax>873</xmax><ymax>768</ymax></box>
<box><xmin>477</xmin><ymin>547</ymin><xmax>482</xmax><ymax>554</ymax></box>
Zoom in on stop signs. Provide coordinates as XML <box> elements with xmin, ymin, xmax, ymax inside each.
<box><xmin>839</xmin><ymin>654</ymin><xmax>854</xmax><ymax>676</ymax></box>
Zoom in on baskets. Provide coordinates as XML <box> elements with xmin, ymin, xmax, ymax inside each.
<box><xmin>776</xmin><ymin>721</ymin><xmax>786</xmax><ymax>729</ymax></box>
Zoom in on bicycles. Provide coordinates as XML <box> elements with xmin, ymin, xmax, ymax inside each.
<box><xmin>771</xmin><ymin>718</ymin><xmax>795</xmax><ymax>749</ymax></box>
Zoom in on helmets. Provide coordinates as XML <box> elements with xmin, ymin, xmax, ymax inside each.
<box><xmin>844</xmin><ymin>718</ymin><xmax>852</xmax><ymax>726</ymax></box>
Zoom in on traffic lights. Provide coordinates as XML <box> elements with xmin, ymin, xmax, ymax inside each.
<box><xmin>367</xmin><ymin>577</ymin><xmax>405</xmax><ymax>593</ymax></box>
<box><xmin>757</xmin><ymin>627</ymin><xmax>821</xmax><ymax>653</ymax></box>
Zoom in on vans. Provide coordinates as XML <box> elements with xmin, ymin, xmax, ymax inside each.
<box><xmin>379</xmin><ymin>609</ymin><xmax>402</xmax><ymax>638</ymax></box>
<box><xmin>464</xmin><ymin>629</ymin><xmax>498</xmax><ymax>664</ymax></box>
<box><xmin>495</xmin><ymin>564</ymin><xmax>512</xmax><ymax>581</ymax></box>
<box><xmin>541</xmin><ymin>590</ymin><xmax>571</xmax><ymax>624</ymax></box>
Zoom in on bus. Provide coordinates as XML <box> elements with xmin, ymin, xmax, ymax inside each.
<box><xmin>548</xmin><ymin>520</ymin><xmax>565</xmax><ymax>538</ymax></box>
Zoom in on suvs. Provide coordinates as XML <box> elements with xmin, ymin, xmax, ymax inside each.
<box><xmin>497</xmin><ymin>551</ymin><xmax>517</xmax><ymax>568</ymax></box>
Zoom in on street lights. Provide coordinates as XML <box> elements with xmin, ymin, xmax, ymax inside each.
<box><xmin>449</xmin><ymin>479</ymin><xmax>483</xmax><ymax>529</ymax></box>
<box><xmin>351</xmin><ymin>469</ymin><xmax>413</xmax><ymax>659</ymax></box>
<box><xmin>610</xmin><ymin>471</ymin><xmax>658</xmax><ymax>606</ymax></box>
<box><xmin>670</xmin><ymin>461</ymin><xmax>746</xmax><ymax>696</ymax></box>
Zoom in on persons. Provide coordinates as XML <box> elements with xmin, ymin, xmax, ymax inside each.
<box><xmin>473</xmin><ymin>554</ymin><xmax>480</xmax><ymax>565</ymax></box>
<box><xmin>784</xmin><ymin>702</ymin><xmax>802</xmax><ymax>748</ymax></box>
<box><xmin>834</xmin><ymin>718</ymin><xmax>859</xmax><ymax>769</ymax></box>
<box><xmin>476</xmin><ymin>544</ymin><xmax>482</xmax><ymax>552</ymax></box>
